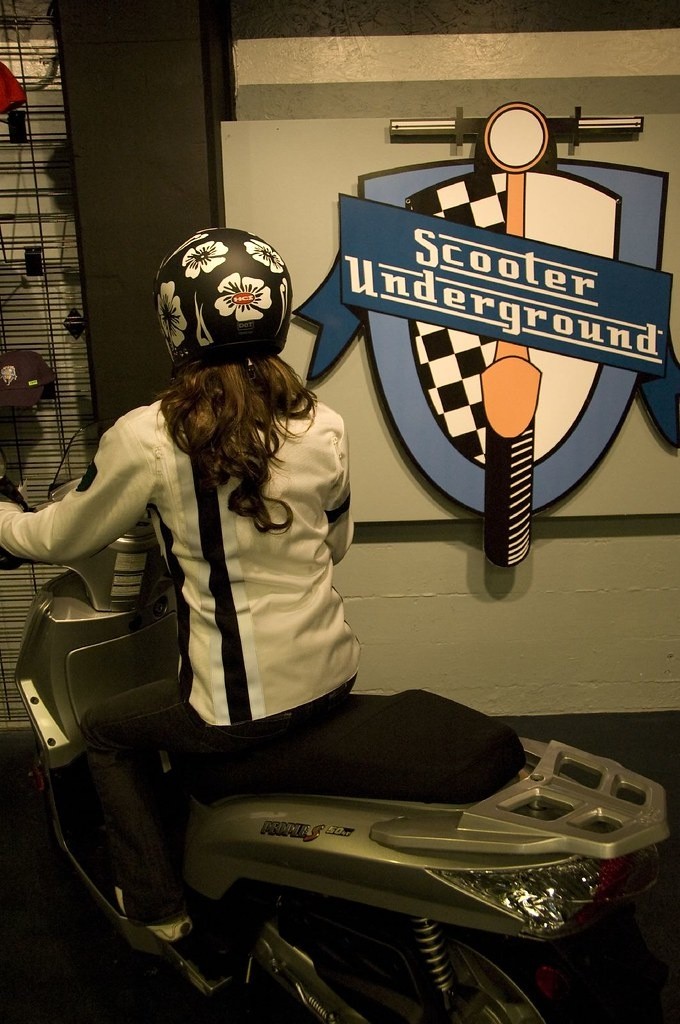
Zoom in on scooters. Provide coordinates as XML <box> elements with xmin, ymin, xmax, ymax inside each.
<box><xmin>0</xmin><ymin>418</ymin><xmax>672</xmax><ymax>1024</ymax></box>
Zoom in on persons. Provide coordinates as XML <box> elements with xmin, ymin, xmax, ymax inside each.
<box><xmin>0</xmin><ymin>228</ymin><xmax>361</xmax><ymax>943</ymax></box>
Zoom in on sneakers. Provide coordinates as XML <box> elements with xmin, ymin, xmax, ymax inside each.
<box><xmin>115</xmin><ymin>886</ymin><xmax>193</xmax><ymax>943</ymax></box>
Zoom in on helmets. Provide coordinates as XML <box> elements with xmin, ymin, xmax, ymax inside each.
<box><xmin>153</xmin><ymin>228</ymin><xmax>293</xmax><ymax>381</ymax></box>
